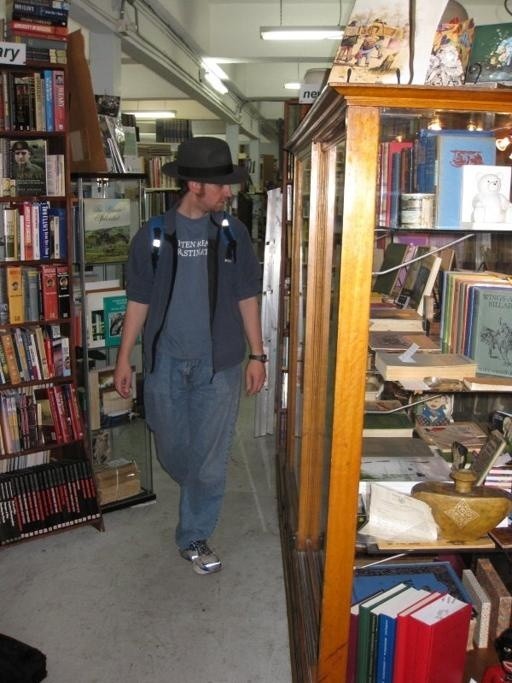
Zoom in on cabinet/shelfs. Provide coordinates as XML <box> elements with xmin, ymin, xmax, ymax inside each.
<box><xmin>274</xmin><ymin>82</ymin><xmax>512</xmax><ymax>683</ymax></box>
<box><xmin>0</xmin><ymin>42</ymin><xmax>180</xmax><ymax>552</ymax></box>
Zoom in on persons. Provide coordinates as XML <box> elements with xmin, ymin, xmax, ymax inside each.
<box><xmin>113</xmin><ymin>136</ymin><xmax>265</xmax><ymax>574</ymax></box>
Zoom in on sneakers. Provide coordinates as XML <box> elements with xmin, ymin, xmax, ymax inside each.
<box><xmin>179</xmin><ymin>540</ymin><xmax>223</xmax><ymax>575</ymax></box>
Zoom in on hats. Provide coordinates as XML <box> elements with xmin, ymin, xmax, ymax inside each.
<box><xmin>9</xmin><ymin>142</ymin><xmax>28</xmax><ymax>152</ymax></box>
<box><xmin>161</xmin><ymin>137</ymin><xmax>248</xmax><ymax>184</ymax></box>
<box><xmin>366</xmin><ymin>23</ymin><xmax>382</xmax><ymax>35</ymax></box>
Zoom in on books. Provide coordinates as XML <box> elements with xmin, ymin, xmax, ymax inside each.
<box><xmin>344</xmin><ymin>129</ymin><xmax>511</xmax><ymax>683</ymax></box>
<box><xmin>0</xmin><ymin>0</ymin><xmax>193</xmax><ymax>546</ymax></box>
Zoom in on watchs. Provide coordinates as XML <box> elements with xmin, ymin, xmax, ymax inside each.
<box><xmin>248</xmin><ymin>354</ymin><xmax>267</xmax><ymax>362</ymax></box>
